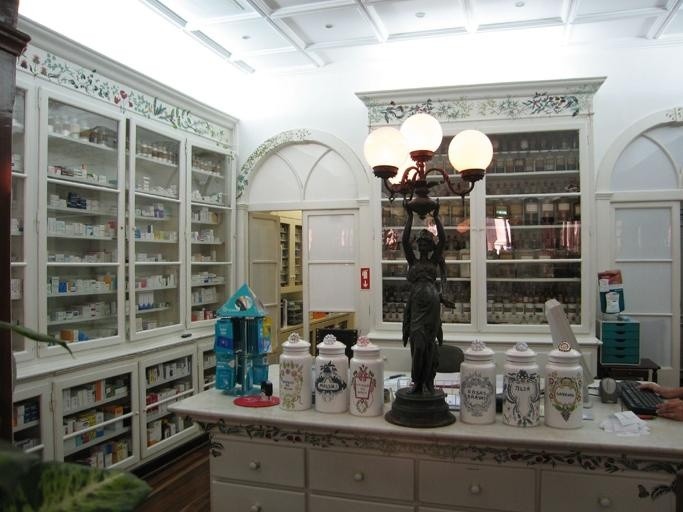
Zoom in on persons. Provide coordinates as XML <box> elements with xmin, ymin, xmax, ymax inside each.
<box><xmin>400</xmin><ymin>198</ymin><xmax>455</xmax><ymax>392</ymax></box>
<box><xmin>635</xmin><ymin>380</ymin><xmax>682</xmax><ymax>421</ymax></box>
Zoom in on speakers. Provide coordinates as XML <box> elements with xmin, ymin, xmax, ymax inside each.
<box><xmin>599</xmin><ymin>376</ymin><xmax>616</xmax><ymax>403</ymax></box>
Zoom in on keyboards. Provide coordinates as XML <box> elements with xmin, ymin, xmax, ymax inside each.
<box><xmin>618</xmin><ymin>377</ymin><xmax>666</xmax><ymax>411</ymax></box>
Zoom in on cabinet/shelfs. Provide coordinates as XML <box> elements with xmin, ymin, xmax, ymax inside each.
<box><xmin>354</xmin><ymin>76</ymin><xmax>607</xmax><ymax>336</ymax></box>
<box><xmin>166</xmin><ymin>367</ymin><xmax>683</xmax><ymax>512</ymax></box>
<box><xmin>275</xmin><ymin>217</ymin><xmax>355</xmax><ymax>358</ymax></box>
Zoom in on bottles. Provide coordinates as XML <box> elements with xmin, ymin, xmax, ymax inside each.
<box><xmin>54</xmin><ymin>113</ymin><xmax>93</xmax><ymax>144</ymax></box>
<box><xmin>350</xmin><ymin>336</ymin><xmax>385</xmax><ymax>417</ymax></box>
<box><xmin>278</xmin><ymin>333</ymin><xmax>312</xmax><ymax>411</ymax></box>
<box><xmin>494</xmin><ymin>149</ymin><xmax>577</xmax><ymax>172</ymax></box>
<box><xmin>137</xmin><ymin>137</ymin><xmax>169</xmax><ymax>163</ymax></box>
<box><xmin>487</xmin><ymin>198</ymin><xmax>580</xmax><ymax>226</ymax></box>
<box><xmin>462</xmin><ymin>302</ymin><xmax>471</xmax><ymax>322</ymax></box>
<box><xmin>459</xmin><ymin>337</ymin><xmax>497</xmax><ymax>425</ymax></box>
<box><xmin>496</xmin><ymin>179</ymin><xmax>556</xmax><ymax>194</ymax></box>
<box><xmin>383</xmin><ymin>303</ymin><xmax>404</xmax><ymax>319</ymax></box>
<box><xmin>502</xmin><ymin>342</ymin><xmax>541</xmax><ymax>428</ymax></box>
<box><xmin>314</xmin><ymin>334</ymin><xmax>349</xmax><ymax>413</ymax></box>
<box><xmin>488</xmin><ymin>296</ymin><xmax>579</xmax><ymax>321</ymax></box>
<box><xmin>80</xmin><ymin>163</ymin><xmax>87</xmax><ymax>178</ymax></box>
<box><xmin>544</xmin><ymin>341</ymin><xmax>584</xmax><ymax>429</ymax></box>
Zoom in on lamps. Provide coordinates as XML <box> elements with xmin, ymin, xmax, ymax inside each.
<box><xmin>362</xmin><ymin>112</ymin><xmax>494</xmax><ymax>221</ymax></box>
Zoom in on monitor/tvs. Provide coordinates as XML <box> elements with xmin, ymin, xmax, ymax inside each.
<box><xmin>543</xmin><ymin>296</ymin><xmax>594</xmax><ymax>408</ymax></box>
